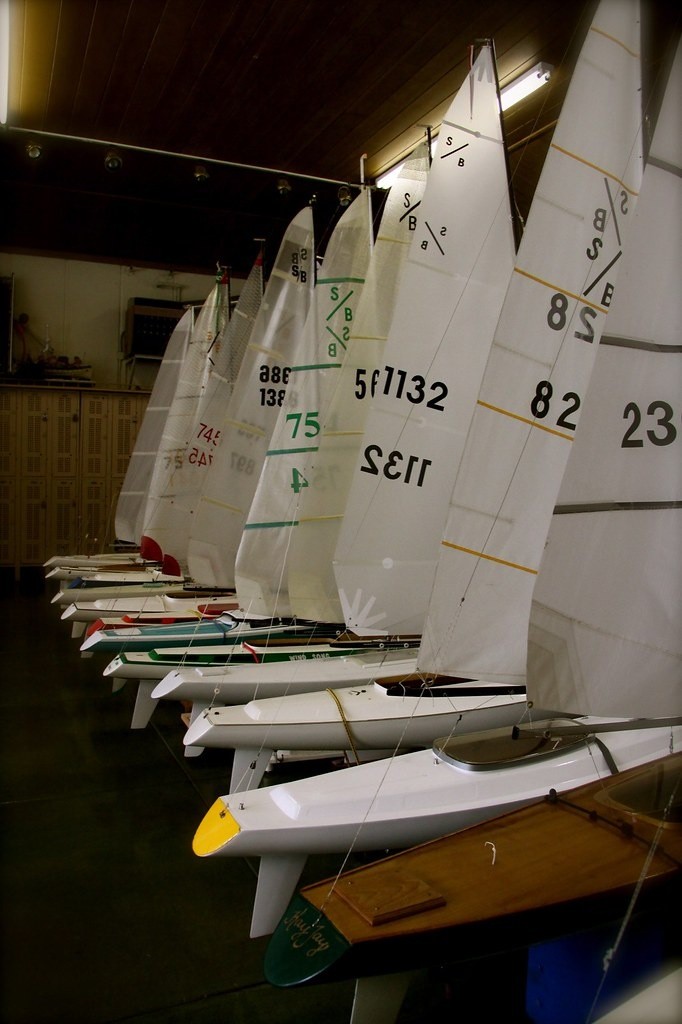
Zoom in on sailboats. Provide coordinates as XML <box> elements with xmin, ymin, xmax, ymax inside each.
<box><xmin>42</xmin><ymin>0</ymin><xmax>682</xmax><ymax>990</ymax></box>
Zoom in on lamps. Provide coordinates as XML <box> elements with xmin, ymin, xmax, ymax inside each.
<box><xmin>276</xmin><ymin>179</ymin><xmax>292</xmax><ymax>196</ymax></box>
<box><xmin>193</xmin><ymin>164</ymin><xmax>212</xmax><ymax>182</ymax></box>
<box><xmin>375</xmin><ymin>62</ymin><xmax>555</xmax><ymax>190</ymax></box>
<box><xmin>102</xmin><ymin>150</ymin><xmax>122</xmax><ymax>172</ymax></box>
<box><xmin>24</xmin><ymin>141</ymin><xmax>43</xmax><ymax>159</ymax></box>
<box><xmin>337</xmin><ymin>185</ymin><xmax>353</xmax><ymax>207</ymax></box>
